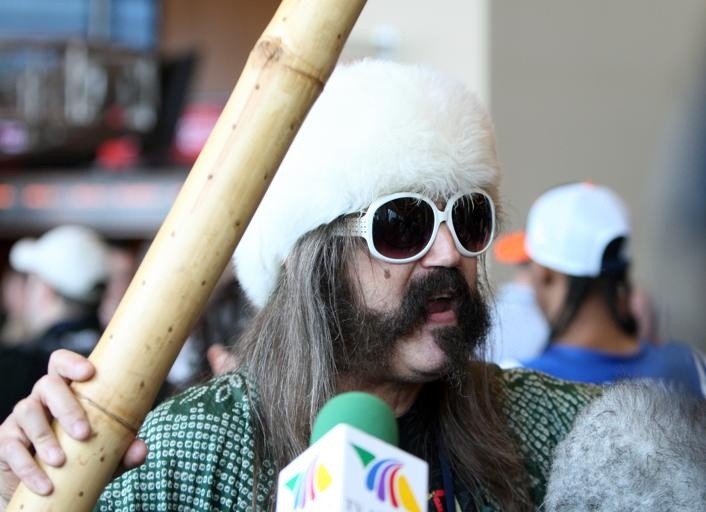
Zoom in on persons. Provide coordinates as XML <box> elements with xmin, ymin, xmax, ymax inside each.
<box><xmin>471</xmin><ymin>230</ymin><xmax>551</xmax><ymax>366</ymax></box>
<box><xmin>0</xmin><ymin>63</ymin><xmax>706</xmax><ymax>512</ymax></box>
<box><xmin>525</xmin><ymin>181</ymin><xmax>706</xmax><ymax>399</ymax></box>
<box><xmin>1</xmin><ymin>224</ymin><xmax>259</xmax><ymax>426</ymax></box>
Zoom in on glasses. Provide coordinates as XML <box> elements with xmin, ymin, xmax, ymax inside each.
<box><xmin>331</xmin><ymin>187</ymin><xmax>497</xmax><ymax>265</ymax></box>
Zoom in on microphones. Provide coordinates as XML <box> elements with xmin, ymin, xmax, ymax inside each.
<box><xmin>309</xmin><ymin>391</ymin><xmax>400</xmax><ymax>512</ymax></box>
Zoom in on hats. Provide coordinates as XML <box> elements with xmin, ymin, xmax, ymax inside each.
<box><xmin>235</xmin><ymin>60</ymin><xmax>500</xmax><ymax>307</ymax></box>
<box><xmin>493</xmin><ymin>184</ymin><xmax>633</xmax><ymax>278</ymax></box>
<box><xmin>8</xmin><ymin>224</ymin><xmax>112</xmax><ymax>304</ymax></box>
